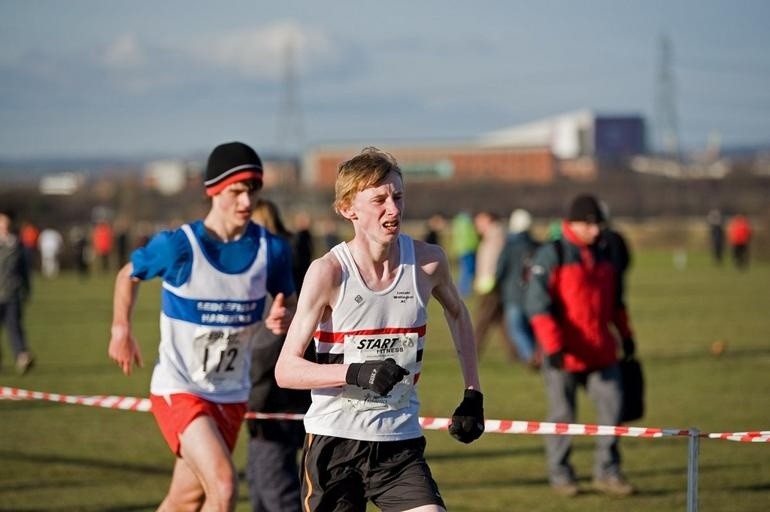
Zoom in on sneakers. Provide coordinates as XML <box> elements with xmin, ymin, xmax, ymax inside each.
<box><xmin>549</xmin><ymin>485</ymin><xmax>582</xmax><ymax>498</ymax></box>
<box><xmin>591</xmin><ymin>476</ymin><xmax>637</xmax><ymax>499</ymax></box>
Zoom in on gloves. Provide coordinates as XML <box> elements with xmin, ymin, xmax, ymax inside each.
<box><xmin>549</xmin><ymin>348</ymin><xmax>568</xmax><ymax>370</ymax></box>
<box><xmin>448</xmin><ymin>388</ymin><xmax>485</xmax><ymax>445</ymax></box>
<box><xmin>622</xmin><ymin>336</ymin><xmax>634</xmax><ymax>356</ymax></box>
<box><xmin>344</xmin><ymin>358</ymin><xmax>404</xmax><ymax>397</ymax></box>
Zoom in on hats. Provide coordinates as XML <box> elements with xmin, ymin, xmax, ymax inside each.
<box><xmin>202</xmin><ymin>142</ymin><xmax>263</xmax><ymax>197</ymax></box>
<box><xmin>568</xmin><ymin>194</ymin><xmax>604</xmax><ymax>223</ymax></box>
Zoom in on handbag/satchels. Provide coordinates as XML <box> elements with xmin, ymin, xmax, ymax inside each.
<box><xmin>619</xmin><ymin>356</ymin><xmax>643</xmax><ymax>421</ymax></box>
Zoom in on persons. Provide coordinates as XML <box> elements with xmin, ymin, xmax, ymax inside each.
<box><xmin>10</xmin><ymin>213</ymin><xmax>155</xmax><ymax>279</ymax></box>
<box><xmin>107</xmin><ymin>142</ymin><xmax>297</xmax><ymax>511</ymax></box>
<box><xmin>291</xmin><ymin>212</ymin><xmax>340</xmax><ymax>280</ymax></box>
<box><xmin>0</xmin><ymin>211</ymin><xmax>34</xmax><ymax>375</ymax></box>
<box><xmin>524</xmin><ymin>196</ymin><xmax>634</xmax><ymax>496</ymax></box>
<box><xmin>246</xmin><ymin>200</ymin><xmax>310</xmax><ymax>511</ymax></box>
<box><xmin>419</xmin><ymin>209</ymin><xmax>564</xmax><ymax>370</ymax></box>
<box><xmin>707</xmin><ymin>209</ymin><xmax>750</xmax><ymax>268</ymax></box>
<box><xmin>273</xmin><ymin>147</ymin><xmax>484</xmax><ymax>511</ymax></box>
